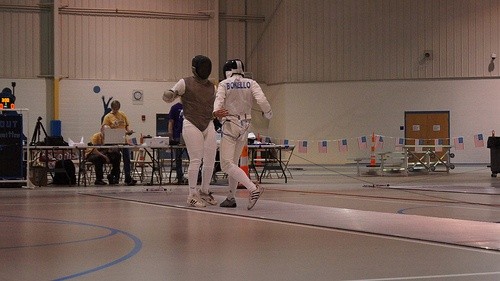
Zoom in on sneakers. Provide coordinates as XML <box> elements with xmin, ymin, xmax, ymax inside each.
<box><xmin>248</xmin><ymin>185</ymin><xmax>264</xmax><ymax>210</ymax></box>
<box><xmin>220</xmin><ymin>198</ymin><xmax>236</xmax><ymax>207</ymax></box>
<box><xmin>187</xmin><ymin>197</ymin><xmax>206</xmax><ymax>207</ymax></box>
<box><xmin>198</xmin><ymin>190</ymin><xmax>217</xmax><ymax>205</ymax></box>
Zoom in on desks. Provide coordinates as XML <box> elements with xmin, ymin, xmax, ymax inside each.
<box><xmin>22</xmin><ymin>144</ymin><xmax>295</xmax><ymax>189</ymax></box>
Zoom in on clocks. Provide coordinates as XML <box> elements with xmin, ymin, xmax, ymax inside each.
<box><xmin>132</xmin><ymin>89</ymin><xmax>143</xmax><ymax>105</ymax></box>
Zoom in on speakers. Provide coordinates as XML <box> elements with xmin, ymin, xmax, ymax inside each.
<box><xmin>0</xmin><ymin>115</ymin><xmax>23</xmax><ymax>180</ymax></box>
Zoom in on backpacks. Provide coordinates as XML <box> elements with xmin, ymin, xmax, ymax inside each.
<box><xmin>52</xmin><ymin>160</ymin><xmax>76</xmax><ymax>185</ymax></box>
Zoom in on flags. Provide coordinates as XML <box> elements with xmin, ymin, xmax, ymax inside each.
<box><xmin>298</xmin><ymin>141</ymin><xmax>307</xmax><ymax>153</ymax></box>
<box><xmin>318</xmin><ymin>140</ymin><xmax>328</xmax><ymax>153</ymax></box>
<box><xmin>375</xmin><ymin>136</ymin><xmax>384</xmax><ymax>149</ymax></box>
<box><xmin>338</xmin><ymin>139</ymin><xmax>348</xmax><ymax>152</ymax></box>
<box><xmin>357</xmin><ymin>136</ymin><xmax>367</xmax><ymax>150</ymax></box>
<box><xmin>393</xmin><ymin>138</ymin><xmax>403</xmax><ymax>151</ymax></box>
<box><xmin>454</xmin><ymin>137</ymin><xmax>464</xmax><ymax>149</ymax></box>
<box><xmin>415</xmin><ymin>139</ymin><xmax>424</xmax><ymax>151</ymax></box>
<box><xmin>474</xmin><ymin>134</ymin><xmax>484</xmax><ymax>147</ymax></box>
<box><xmin>435</xmin><ymin>140</ymin><xmax>442</xmax><ymax>152</ymax></box>
<box><xmin>262</xmin><ymin>136</ymin><xmax>271</xmax><ymax>143</ymax></box>
<box><xmin>279</xmin><ymin>139</ymin><xmax>288</xmax><ymax>145</ymax></box>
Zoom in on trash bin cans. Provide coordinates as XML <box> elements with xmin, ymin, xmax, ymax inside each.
<box><xmin>487</xmin><ymin>136</ymin><xmax>500</xmax><ymax>177</ymax></box>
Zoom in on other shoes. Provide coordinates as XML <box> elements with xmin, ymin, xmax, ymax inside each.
<box><xmin>125</xmin><ymin>180</ymin><xmax>137</xmax><ymax>186</ymax></box>
<box><xmin>178</xmin><ymin>178</ymin><xmax>188</xmax><ymax>185</ymax></box>
<box><xmin>107</xmin><ymin>174</ymin><xmax>115</xmax><ymax>184</ymax></box>
<box><xmin>115</xmin><ymin>177</ymin><xmax>119</xmax><ymax>184</ymax></box>
<box><xmin>94</xmin><ymin>180</ymin><xmax>107</xmax><ymax>185</ymax></box>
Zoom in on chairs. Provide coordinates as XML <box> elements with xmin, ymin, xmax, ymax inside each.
<box><xmin>29</xmin><ymin>141</ymin><xmax>296</xmax><ymax>189</ymax></box>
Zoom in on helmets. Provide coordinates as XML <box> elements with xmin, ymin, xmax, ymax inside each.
<box><xmin>192</xmin><ymin>55</ymin><xmax>212</xmax><ymax>79</ymax></box>
<box><xmin>223</xmin><ymin>59</ymin><xmax>245</xmax><ymax>79</ymax></box>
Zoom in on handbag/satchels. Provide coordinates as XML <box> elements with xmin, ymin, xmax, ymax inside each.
<box><xmin>44</xmin><ymin>136</ymin><xmax>69</xmax><ymax>146</ymax></box>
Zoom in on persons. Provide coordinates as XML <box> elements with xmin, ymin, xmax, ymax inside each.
<box><xmin>102</xmin><ymin>100</ymin><xmax>137</xmax><ymax>186</ymax></box>
<box><xmin>168</xmin><ymin>97</ymin><xmax>202</xmax><ymax>184</ymax></box>
<box><xmin>212</xmin><ymin>59</ymin><xmax>273</xmax><ymax>211</ymax></box>
<box><xmin>85</xmin><ymin>124</ymin><xmax>115</xmax><ymax>185</ymax></box>
<box><xmin>162</xmin><ymin>55</ymin><xmax>217</xmax><ymax>207</ymax></box>
